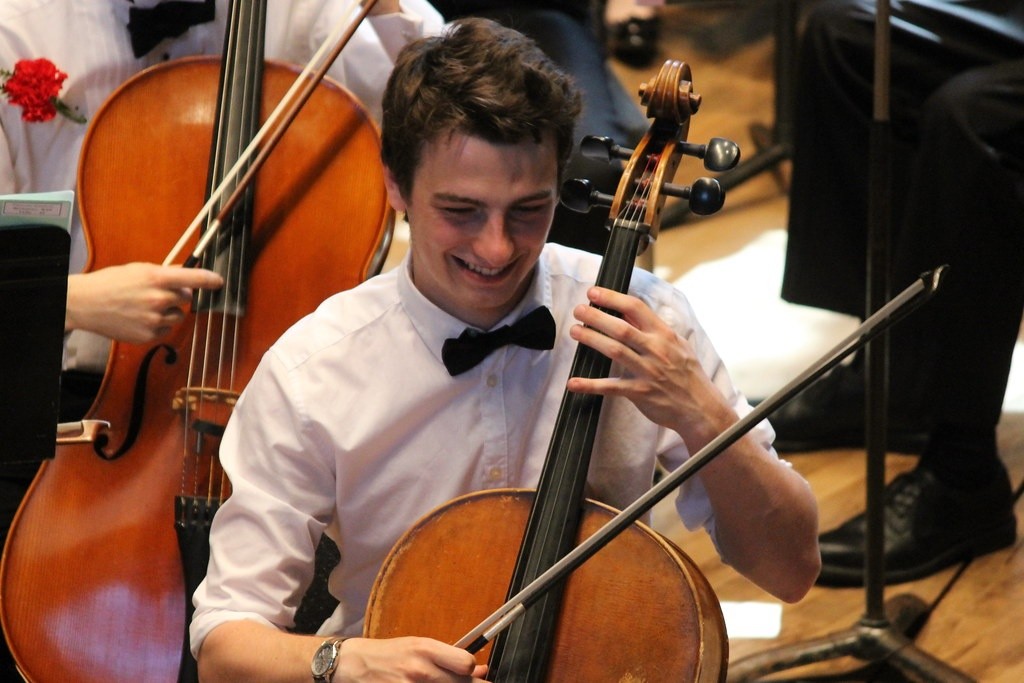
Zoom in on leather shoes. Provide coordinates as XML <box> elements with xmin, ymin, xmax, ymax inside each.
<box><xmin>740</xmin><ymin>365</ymin><xmax>924</xmax><ymax>456</ymax></box>
<box><xmin>817</xmin><ymin>472</ymin><xmax>1016</xmax><ymax>588</ymax></box>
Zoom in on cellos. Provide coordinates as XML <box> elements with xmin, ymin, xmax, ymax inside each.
<box><xmin>0</xmin><ymin>1</ymin><xmax>397</xmax><ymax>682</ymax></box>
<box><xmin>353</xmin><ymin>59</ymin><xmax>742</xmax><ymax>683</ymax></box>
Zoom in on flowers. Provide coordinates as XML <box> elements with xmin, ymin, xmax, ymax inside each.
<box><xmin>0</xmin><ymin>57</ymin><xmax>88</xmax><ymax>127</ymax></box>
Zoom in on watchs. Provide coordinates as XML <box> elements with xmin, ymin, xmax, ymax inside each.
<box><xmin>311</xmin><ymin>635</ymin><xmax>351</xmax><ymax>683</ymax></box>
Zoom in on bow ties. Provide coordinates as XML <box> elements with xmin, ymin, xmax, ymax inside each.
<box><xmin>442</xmin><ymin>305</ymin><xmax>556</xmax><ymax>376</ymax></box>
<box><xmin>127</xmin><ymin>0</ymin><xmax>216</xmax><ymax>59</ymax></box>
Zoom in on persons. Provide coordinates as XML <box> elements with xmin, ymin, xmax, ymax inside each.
<box><xmin>770</xmin><ymin>0</ymin><xmax>1024</xmax><ymax>590</ymax></box>
<box><xmin>187</xmin><ymin>15</ymin><xmax>823</xmax><ymax>683</ymax></box>
<box><xmin>0</xmin><ymin>0</ymin><xmax>450</xmax><ymax>428</ymax></box>
